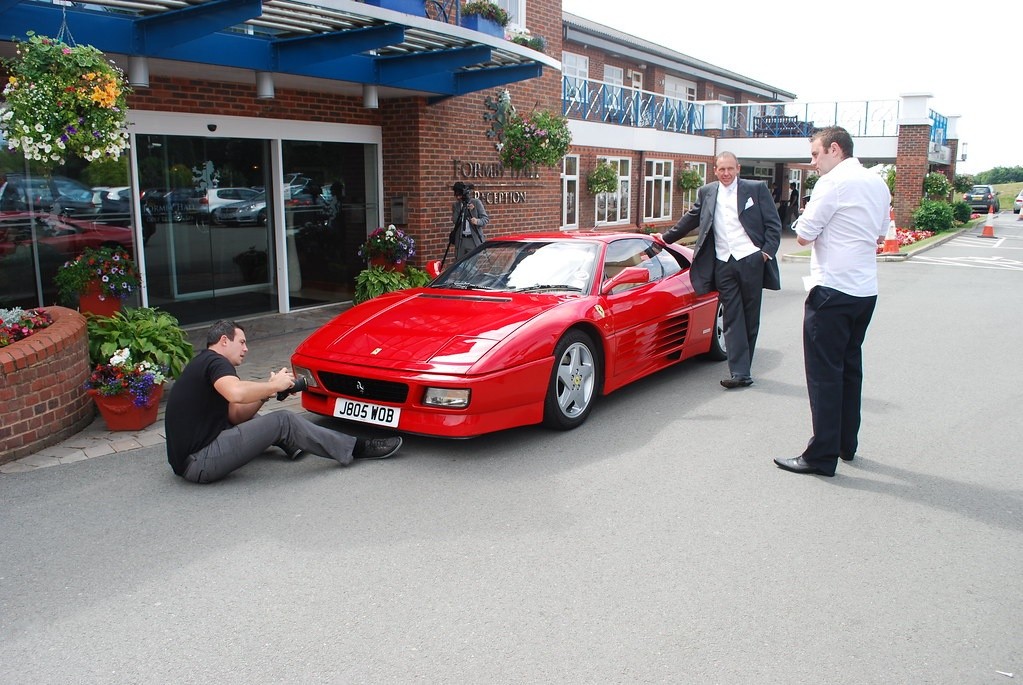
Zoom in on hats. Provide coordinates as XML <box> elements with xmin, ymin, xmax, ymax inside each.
<box><xmin>453</xmin><ymin>182</ymin><xmax>465</xmax><ymax>196</ymax></box>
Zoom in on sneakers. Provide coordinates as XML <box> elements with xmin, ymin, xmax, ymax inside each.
<box><xmin>353</xmin><ymin>436</ymin><xmax>403</xmax><ymax>459</ymax></box>
<box><xmin>287</xmin><ymin>448</ymin><xmax>302</xmax><ymax>459</ymax></box>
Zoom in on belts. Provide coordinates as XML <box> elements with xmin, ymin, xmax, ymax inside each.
<box><xmin>462</xmin><ymin>234</ymin><xmax>472</xmax><ymax>238</ymax></box>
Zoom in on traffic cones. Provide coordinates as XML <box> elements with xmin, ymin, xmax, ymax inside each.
<box><xmin>1016</xmin><ymin>207</ymin><xmax>1023</xmax><ymax>221</ymax></box>
<box><xmin>977</xmin><ymin>205</ymin><xmax>997</xmax><ymax>238</ymax></box>
<box><xmin>876</xmin><ymin>210</ymin><xmax>909</xmax><ymax>256</ymax></box>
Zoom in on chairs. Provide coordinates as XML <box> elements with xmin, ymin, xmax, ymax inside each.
<box><xmin>603</xmin><ymin>247</ymin><xmax>648</xmax><ymax>293</ymax></box>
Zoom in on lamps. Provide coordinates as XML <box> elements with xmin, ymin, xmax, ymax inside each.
<box><xmin>363</xmin><ymin>85</ymin><xmax>378</xmax><ymax>109</ymax></box>
<box><xmin>709</xmin><ymin>91</ymin><xmax>714</xmax><ymax>99</ymax></box>
<box><xmin>926</xmin><ymin>129</ymin><xmax>944</xmax><ymax>156</ymax></box>
<box><xmin>256</xmin><ymin>70</ymin><xmax>275</xmax><ymax>100</ymax></box>
<box><xmin>638</xmin><ymin>64</ymin><xmax>646</xmax><ymax>69</ymax></box>
<box><xmin>954</xmin><ymin>143</ymin><xmax>968</xmax><ymax>163</ymax></box>
<box><xmin>128</xmin><ymin>56</ymin><xmax>150</xmax><ymax>88</ymax></box>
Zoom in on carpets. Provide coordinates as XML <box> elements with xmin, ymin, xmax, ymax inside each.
<box><xmin>149</xmin><ymin>290</ymin><xmax>328</xmax><ymax>327</ymax></box>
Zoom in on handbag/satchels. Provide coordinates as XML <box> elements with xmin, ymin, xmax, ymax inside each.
<box><xmin>449</xmin><ymin>230</ymin><xmax>455</xmax><ymax>244</ymax></box>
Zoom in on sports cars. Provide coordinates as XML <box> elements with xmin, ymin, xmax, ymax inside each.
<box><xmin>291</xmin><ymin>231</ymin><xmax>728</xmax><ymax>441</ymax></box>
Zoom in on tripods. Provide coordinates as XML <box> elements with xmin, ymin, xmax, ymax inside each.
<box><xmin>438</xmin><ymin>197</ymin><xmax>492</xmax><ymax>273</ymax></box>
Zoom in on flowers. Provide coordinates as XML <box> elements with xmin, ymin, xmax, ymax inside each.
<box><xmin>83</xmin><ymin>348</ymin><xmax>169</xmax><ymax>407</ymax></box>
<box><xmin>495</xmin><ymin>105</ymin><xmax>574</xmax><ymax>175</ymax></box>
<box><xmin>0</xmin><ymin>29</ymin><xmax>137</xmax><ymax>179</ymax></box>
<box><xmin>586</xmin><ymin>159</ymin><xmax>618</xmax><ymax>195</ymax></box>
<box><xmin>358</xmin><ymin>224</ymin><xmax>417</xmax><ymax>265</ymax></box>
<box><xmin>678</xmin><ymin>166</ymin><xmax>705</xmax><ymax>191</ymax></box>
<box><xmin>54</xmin><ymin>242</ymin><xmax>143</xmax><ymax>303</ymax></box>
<box><xmin>462</xmin><ymin>0</ymin><xmax>550</xmax><ymax>54</ymax></box>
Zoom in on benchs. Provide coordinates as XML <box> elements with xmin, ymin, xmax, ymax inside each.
<box><xmin>752</xmin><ymin>115</ymin><xmax>803</xmax><ymax>137</ymax></box>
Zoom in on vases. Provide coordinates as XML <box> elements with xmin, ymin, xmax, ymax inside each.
<box><xmin>93</xmin><ymin>381</ymin><xmax>164</xmax><ymax>431</ymax></box>
<box><xmin>461</xmin><ymin>13</ymin><xmax>504</xmax><ymax>39</ymax></box>
<box><xmin>365</xmin><ymin>0</ymin><xmax>426</xmax><ymax>16</ymax></box>
<box><xmin>79</xmin><ymin>281</ymin><xmax>120</xmax><ymax>327</ymax></box>
<box><xmin>370</xmin><ymin>255</ymin><xmax>406</xmax><ymax>274</ymax></box>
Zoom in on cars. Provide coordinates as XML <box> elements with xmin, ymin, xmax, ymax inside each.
<box><xmin>962</xmin><ymin>184</ymin><xmax>1000</xmax><ymax>213</ymax></box>
<box><xmin>0</xmin><ymin>212</ymin><xmax>138</xmax><ymax>269</ymax></box>
<box><xmin>1013</xmin><ymin>189</ymin><xmax>1023</xmax><ymax>213</ymax></box>
<box><xmin>94</xmin><ymin>173</ymin><xmax>332</xmax><ymax>229</ymax></box>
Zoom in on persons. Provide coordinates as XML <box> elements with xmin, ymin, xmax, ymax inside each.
<box><xmin>451</xmin><ymin>182</ymin><xmax>490</xmax><ymax>275</ymax></box>
<box><xmin>650</xmin><ymin>151</ymin><xmax>782</xmax><ymax>389</ymax></box>
<box><xmin>165</xmin><ymin>319</ymin><xmax>403</xmax><ymax>483</ymax></box>
<box><xmin>785</xmin><ymin>183</ymin><xmax>799</xmax><ymax>219</ymax></box>
<box><xmin>772</xmin><ymin>182</ymin><xmax>781</xmax><ymax>209</ymax></box>
<box><xmin>773</xmin><ymin>125</ymin><xmax>891</xmax><ymax>477</ymax></box>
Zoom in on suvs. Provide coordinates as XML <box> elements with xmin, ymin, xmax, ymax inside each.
<box><xmin>0</xmin><ymin>172</ymin><xmax>156</xmax><ymax>244</ymax></box>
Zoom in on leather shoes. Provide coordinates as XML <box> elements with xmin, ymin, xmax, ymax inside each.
<box><xmin>720</xmin><ymin>374</ymin><xmax>753</xmax><ymax>388</ymax></box>
<box><xmin>774</xmin><ymin>455</ymin><xmax>825</xmax><ymax>475</ymax></box>
